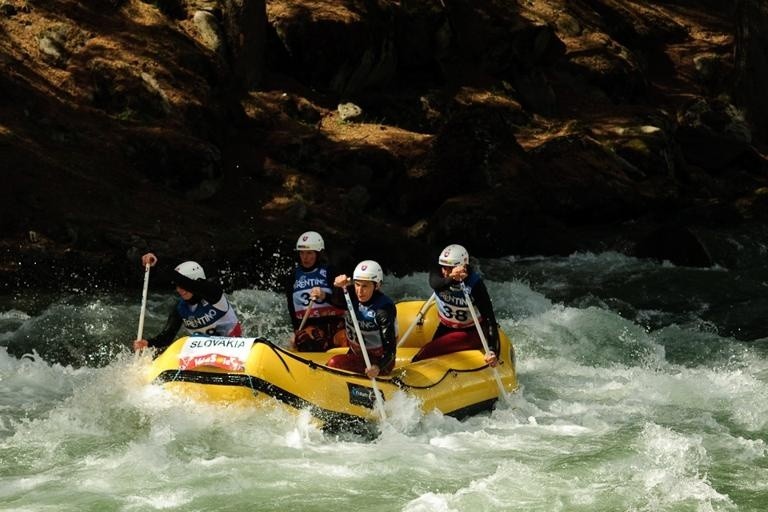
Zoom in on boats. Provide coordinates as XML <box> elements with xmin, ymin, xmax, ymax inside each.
<box><xmin>139</xmin><ymin>300</ymin><xmax>516</xmax><ymax>435</ymax></box>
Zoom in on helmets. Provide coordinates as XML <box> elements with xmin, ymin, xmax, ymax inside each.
<box><xmin>296</xmin><ymin>231</ymin><xmax>325</xmax><ymax>252</ymax></box>
<box><xmin>438</xmin><ymin>244</ymin><xmax>469</xmax><ymax>267</ymax></box>
<box><xmin>174</xmin><ymin>262</ymin><xmax>207</xmax><ymax>281</ymax></box>
<box><xmin>353</xmin><ymin>260</ymin><xmax>384</xmax><ymax>284</ymax></box>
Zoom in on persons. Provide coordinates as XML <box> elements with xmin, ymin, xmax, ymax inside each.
<box><xmin>285</xmin><ymin>231</ymin><xmax>348</xmax><ymax>353</ymax></box>
<box><xmin>132</xmin><ymin>252</ymin><xmax>242</xmax><ymax>354</ymax></box>
<box><xmin>410</xmin><ymin>243</ymin><xmax>500</xmax><ymax>368</ymax></box>
<box><xmin>326</xmin><ymin>260</ymin><xmax>399</xmax><ymax>379</ymax></box>
